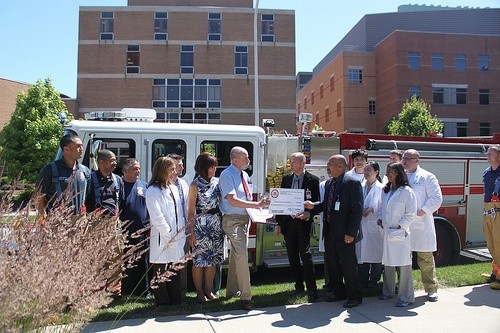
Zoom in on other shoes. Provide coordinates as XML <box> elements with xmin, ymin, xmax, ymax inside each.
<box><xmin>490</xmin><ymin>280</ymin><xmax>500</xmax><ymax>290</ymax></box>
<box><xmin>396</xmin><ymin>300</ymin><xmax>411</xmax><ymax>307</ymax></box>
<box><xmin>292</xmin><ymin>288</ymin><xmax>305</xmax><ymax>294</ymax></box>
<box><xmin>378</xmin><ymin>295</ymin><xmax>391</xmax><ymax>300</ymax></box>
<box><xmin>427</xmin><ymin>292</ymin><xmax>438</xmax><ymax>301</ymax></box>
<box><xmin>328</xmin><ymin>294</ymin><xmax>347</xmax><ymax>301</ymax></box>
<box><xmin>343</xmin><ymin>298</ymin><xmax>362</xmax><ymax>307</ymax></box>
<box><xmin>197</xmin><ymin>290</ymin><xmax>217</xmax><ymax>302</ymax></box>
<box><xmin>307</xmin><ymin>294</ymin><xmax>319</xmax><ymax>302</ymax></box>
<box><xmin>241</xmin><ymin>300</ymin><xmax>253</xmax><ymax>309</ymax></box>
<box><xmin>481</xmin><ymin>274</ymin><xmax>496</xmax><ymax>284</ymax></box>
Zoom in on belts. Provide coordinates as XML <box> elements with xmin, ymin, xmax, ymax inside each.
<box><xmin>483</xmin><ymin>207</ymin><xmax>500</xmax><ymax>215</ymax></box>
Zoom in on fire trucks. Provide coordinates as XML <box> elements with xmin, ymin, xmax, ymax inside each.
<box><xmin>54</xmin><ymin>108</ymin><xmax>500</xmax><ymax>289</ymax></box>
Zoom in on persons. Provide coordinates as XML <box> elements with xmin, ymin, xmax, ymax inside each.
<box><xmin>483</xmin><ymin>144</ymin><xmax>500</xmax><ymax>287</ymax></box>
<box><xmin>38</xmin><ymin>133</ymin><xmax>89</xmax><ymax>220</ymax></box>
<box><xmin>119</xmin><ymin>158</ymin><xmax>150</xmax><ymax>296</ymax></box>
<box><xmin>187</xmin><ymin>150</ymin><xmax>225</xmax><ymax>305</ymax></box>
<box><xmin>389</xmin><ymin>149</ymin><xmax>444</xmax><ymax>302</ymax></box>
<box><xmin>346</xmin><ymin>149</ymin><xmax>369</xmax><ymax>182</ymax></box>
<box><xmin>219</xmin><ymin>146</ymin><xmax>271</xmax><ymax>310</ymax></box>
<box><xmin>376</xmin><ymin>162</ymin><xmax>417</xmax><ymax>307</ymax></box>
<box><xmin>86</xmin><ymin>150</ymin><xmax>124</xmax><ymax>217</ymax></box>
<box><xmin>145</xmin><ymin>152</ymin><xmax>189</xmax><ymax>307</ymax></box>
<box><xmin>274</xmin><ymin>152</ymin><xmax>321</xmax><ymax>303</ymax></box>
<box><xmin>355</xmin><ymin>160</ymin><xmax>384</xmax><ymax>298</ymax></box>
<box><xmin>303</xmin><ymin>154</ymin><xmax>364</xmax><ymax>308</ymax></box>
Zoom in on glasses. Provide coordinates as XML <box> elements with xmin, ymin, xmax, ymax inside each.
<box><xmin>110</xmin><ymin>159</ymin><xmax>117</xmax><ymax>164</ymax></box>
<box><xmin>384</xmin><ymin>169</ymin><xmax>394</xmax><ymax>175</ymax></box>
<box><xmin>76</xmin><ymin>144</ymin><xmax>83</xmax><ymax>148</ymax></box>
<box><xmin>401</xmin><ymin>156</ymin><xmax>417</xmax><ymax>161</ymax></box>
<box><xmin>172</xmin><ymin>170</ymin><xmax>175</xmax><ymax>174</ymax></box>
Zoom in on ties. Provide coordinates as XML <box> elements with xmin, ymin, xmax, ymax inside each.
<box><xmin>240</xmin><ymin>170</ymin><xmax>252</xmax><ymax>201</ymax></box>
<box><xmin>326</xmin><ymin>178</ymin><xmax>337</xmax><ymax>223</ymax></box>
<box><xmin>293</xmin><ymin>177</ymin><xmax>299</xmax><ymax>190</ymax></box>
